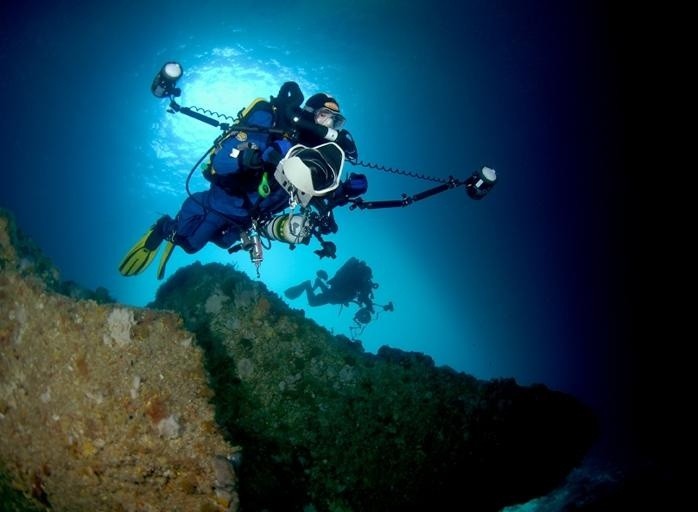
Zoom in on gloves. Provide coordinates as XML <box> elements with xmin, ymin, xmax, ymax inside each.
<box><xmin>261</xmin><ymin>140</ymin><xmax>293</xmax><ymax>167</ymax></box>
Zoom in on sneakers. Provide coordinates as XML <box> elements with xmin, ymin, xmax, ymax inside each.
<box><xmin>145</xmin><ymin>215</ymin><xmax>170</xmax><ymax>250</ymax></box>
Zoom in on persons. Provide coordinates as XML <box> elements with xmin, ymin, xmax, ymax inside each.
<box><xmin>145</xmin><ymin>91</ymin><xmax>357</xmax><ymax>258</ymax></box>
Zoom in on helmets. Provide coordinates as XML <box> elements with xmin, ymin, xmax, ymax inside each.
<box><xmin>302</xmin><ymin>92</ymin><xmax>346</xmax><ymax>131</ymax></box>
